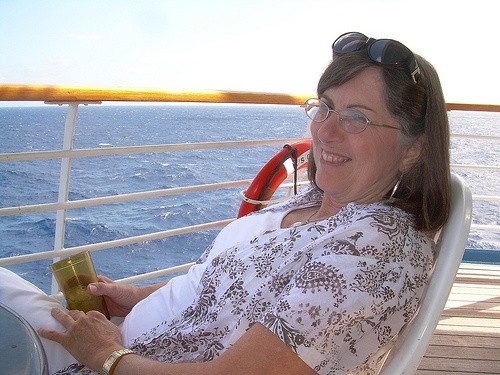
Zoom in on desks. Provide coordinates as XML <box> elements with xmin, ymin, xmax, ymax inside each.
<box><xmin>0</xmin><ymin>301</ymin><xmax>50</xmax><ymax>375</ymax></box>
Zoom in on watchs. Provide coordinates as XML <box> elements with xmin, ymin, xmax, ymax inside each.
<box><xmin>101</xmin><ymin>348</ymin><xmax>136</xmax><ymax>375</ymax></box>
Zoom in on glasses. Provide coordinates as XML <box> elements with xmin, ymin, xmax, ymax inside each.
<box><xmin>331</xmin><ymin>32</ymin><xmax>424</xmax><ymax>87</ymax></box>
<box><xmin>300</xmin><ymin>98</ymin><xmax>405</xmax><ymax>134</ymax></box>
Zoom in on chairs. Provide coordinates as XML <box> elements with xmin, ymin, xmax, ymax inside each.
<box><xmin>372</xmin><ymin>171</ymin><xmax>473</xmax><ymax>375</ymax></box>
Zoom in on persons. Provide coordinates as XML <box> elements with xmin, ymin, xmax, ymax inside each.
<box><xmin>0</xmin><ymin>31</ymin><xmax>452</xmax><ymax>375</ymax></box>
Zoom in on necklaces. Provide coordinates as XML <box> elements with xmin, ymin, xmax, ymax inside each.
<box><xmin>306</xmin><ymin>210</ymin><xmax>327</xmax><ymax>222</ymax></box>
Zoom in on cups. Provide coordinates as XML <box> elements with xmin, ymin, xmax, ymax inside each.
<box><xmin>49</xmin><ymin>250</ymin><xmax>110</xmax><ymax>320</ymax></box>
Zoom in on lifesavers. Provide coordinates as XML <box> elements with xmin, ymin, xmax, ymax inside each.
<box><xmin>237</xmin><ymin>138</ymin><xmax>315</xmax><ymax>220</ymax></box>
